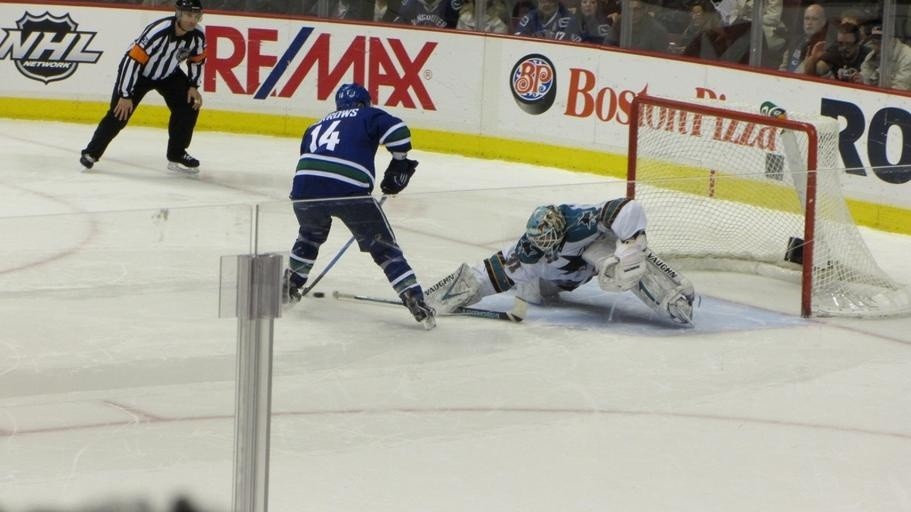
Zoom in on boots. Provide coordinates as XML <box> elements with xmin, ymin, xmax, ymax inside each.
<box><xmin>282</xmin><ymin>267</ymin><xmax>308</xmax><ymax>302</ymax></box>
<box><xmin>80</xmin><ymin>149</ymin><xmax>99</xmax><ymax>168</ymax></box>
<box><xmin>399</xmin><ymin>284</ymin><xmax>437</xmax><ymax>322</ymax></box>
<box><xmin>166</xmin><ymin>148</ymin><xmax>199</xmax><ymax>167</ymax></box>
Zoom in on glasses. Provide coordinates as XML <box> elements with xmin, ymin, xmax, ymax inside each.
<box><xmin>836</xmin><ymin>40</ymin><xmax>858</xmax><ymax>50</ymax></box>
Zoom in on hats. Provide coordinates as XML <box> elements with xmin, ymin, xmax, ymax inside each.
<box><xmin>868</xmin><ymin>22</ymin><xmax>886</xmax><ymax>45</ymax></box>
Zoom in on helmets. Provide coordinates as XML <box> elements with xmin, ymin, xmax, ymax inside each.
<box><xmin>335</xmin><ymin>83</ymin><xmax>371</xmax><ymax>110</ymax></box>
<box><xmin>174</xmin><ymin>0</ymin><xmax>204</xmax><ymax>14</ymax></box>
<box><xmin>525</xmin><ymin>205</ymin><xmax>567</xmax><ymax>254</ymax></box>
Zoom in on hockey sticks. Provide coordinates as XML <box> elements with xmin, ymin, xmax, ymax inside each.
<box><xmin>334</xmin><ymin>282</ymin><xmax>528</xmax><ymax>323</ymax></box>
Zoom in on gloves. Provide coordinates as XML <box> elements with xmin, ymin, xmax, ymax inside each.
<box><xmin>379</xmin><ymin>157</ymin><xmax>418</xmax><ymax>196</ymax></box>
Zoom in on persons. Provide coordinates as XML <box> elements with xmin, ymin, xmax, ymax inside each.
<box><xmin>435</xmin><ymin>198</ymin><xmax>700</xmax><ymax>322</ymax></box>
<box><xmin>81</xmin><ymin>0</ymin><xmax>207</xmax><ymax>170</ymax></box>
<box><xmin>306</xmin><ymin>0</ymin><xmax>910</xmax><ymax>93</ymax></box>
<box><xmin>281</xmin><ymin>84</ymin><xmax>432</xmax><ymax>321</ymax></box>
